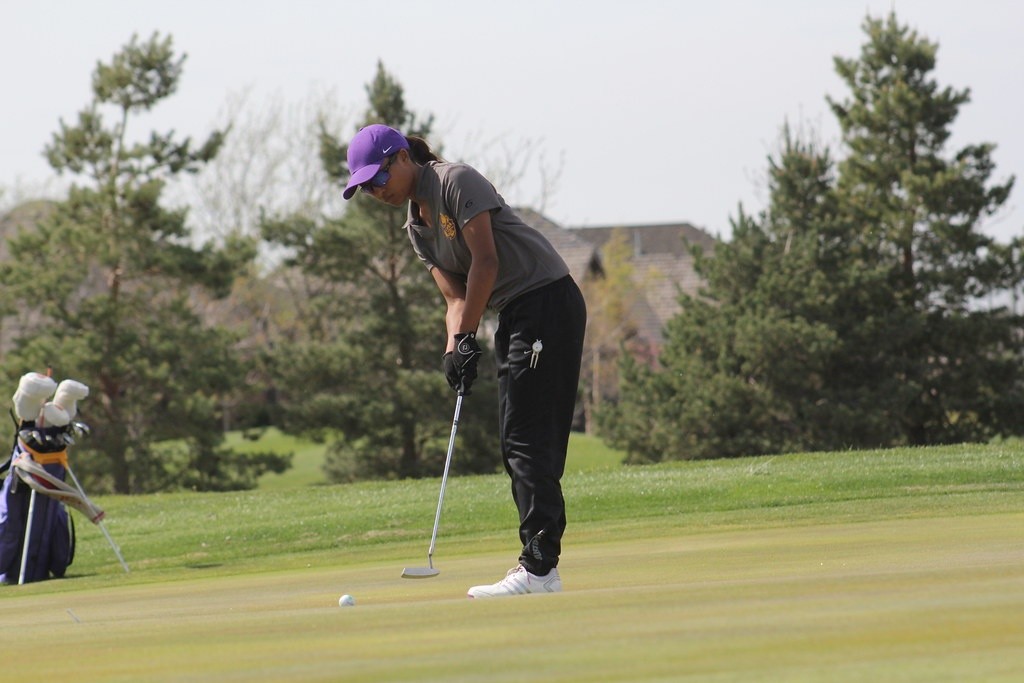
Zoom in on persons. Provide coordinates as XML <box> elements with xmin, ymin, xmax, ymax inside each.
<box><xmin>342</xmin><ymin>124</ymin><xmax>587</xmax><ymax>597</ymax></box>
<box><xmin>0</xmin><ymin>373</ymin><xmax>89</xmax><ymax>585</ymax></box>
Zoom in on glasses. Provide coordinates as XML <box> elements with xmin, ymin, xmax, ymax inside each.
<box><xmin>360</xmin><ymin>152</ymin><xmax>397</xmax><ymax>194</ymax></box>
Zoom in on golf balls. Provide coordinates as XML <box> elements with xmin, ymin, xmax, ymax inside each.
<box><xmin>339</xmin><ymin>594</ymin><xmax>355</xmax><ymax>607</ymax></box>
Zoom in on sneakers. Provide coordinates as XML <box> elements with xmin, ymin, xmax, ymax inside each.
<box><xmin>468</xmin><ymin>564</ymin><xmax>562</xmax><ymax>598</ymax></box>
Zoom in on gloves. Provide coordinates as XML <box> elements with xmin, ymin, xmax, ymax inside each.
<box><xmin>442</xmin><ymin>351</ymin><xmax>478</xmax><ymax>396</ymax></box>
<box><xmin>451</xmin><ymin>331</ymin><xmax>482</xmax><ymax>383</ymax></box>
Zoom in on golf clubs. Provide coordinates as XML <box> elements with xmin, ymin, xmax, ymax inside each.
<box><xmin>400</xmin><ymin>381</ymin><xmax>466</xmax><ymax>580</ymax></box>
<box><xmin>12</xmin><ymin>371</ymin><xmax>91</xmax><ymax>448</ymax></box>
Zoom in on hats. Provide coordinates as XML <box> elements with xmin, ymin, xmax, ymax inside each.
<box><xmin>343</xmin><ymin>124</ymin><xmax>411</xmax><ymax>199</ymax></box>
